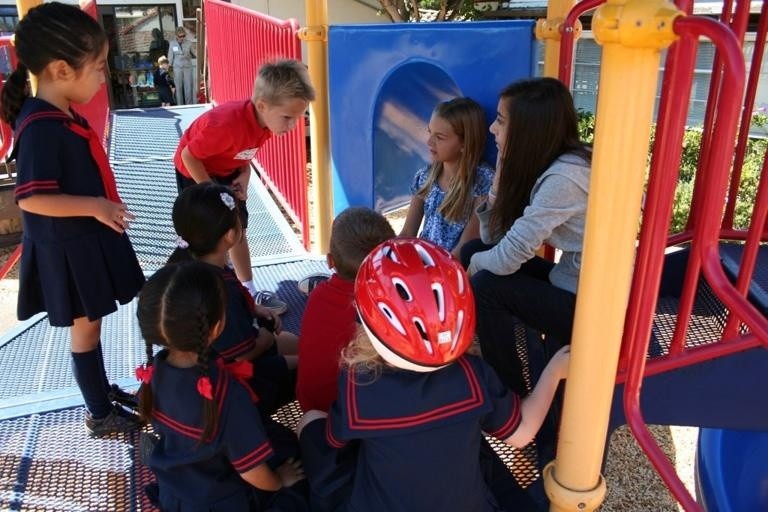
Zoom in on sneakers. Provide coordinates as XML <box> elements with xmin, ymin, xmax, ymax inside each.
<box><xmin>298</xmin><ymin>272</ymin><xmax>332</xmax><ymax>296</ymax></box>
<box><xmin>253</xmin><ymin>290</ymin><xmax>288</xmax><ymax>314</ymax></box>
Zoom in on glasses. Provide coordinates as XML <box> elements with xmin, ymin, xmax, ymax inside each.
<box><xmin>178</xmin><ymin>34</ymin><xmax>185</xmax><ymax>38</ymax></box>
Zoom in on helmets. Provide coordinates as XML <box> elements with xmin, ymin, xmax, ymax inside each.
<box><xmin>354</xmin><ymin>237</ymin><xmax>476</xmax><ymax>367</ymax></box>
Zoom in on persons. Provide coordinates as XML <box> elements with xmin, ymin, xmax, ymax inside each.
<box><xmin>153</xmin><ymin>55</ymin><xmax>176</xmax><ymax>107</ymax></box>
<box><xmin>397</xmin><ymin>95</ymin><xmax>496</xmax><ymax>262</ymax></box>
<box><xmin>136</xmin><ymin>261</ymin><xmax>306</xmax><ymax>512</ymax></box>
<box><xmin>167</xmin><ymin>26</ymin><xmax>197</xmax><ymax>105</ymax></box>
<box><xmin>297</xmin><ymin>237</ymin><xmax>571</xmax><ymax>512</ymax></box>
<box><xmin>460</xmin><ymin>76</ymin><xmax>593</xmax><ymax>403</ymax></box>
<box><xmin>173</xmin><ymin>59</ymin><xmax>315</xmax><ymax>315</ymax></box>
<box><xmin>296</xmin><ymin>205</ymin><xmax>398</xmax><ymax>415</ymax></box>
<box><xmin>165</xmin><ymin>180</ymin><xmax>300</xmax><ymax>393</ymax></box>
<box><xmin>0</xmin><ymin>1</ymin><xmax>147</xmax><ymax>436</ymax></box>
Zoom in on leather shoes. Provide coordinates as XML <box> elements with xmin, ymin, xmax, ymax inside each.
<box><xmin>108</xmin><ymin>383</ymin><xmax>140</xmax><ymax>407</ymax></box>
<box><xmin>85</xmin><ymin>401</ymin><xmax>144</xmax><ymax>435</ymax></box>
